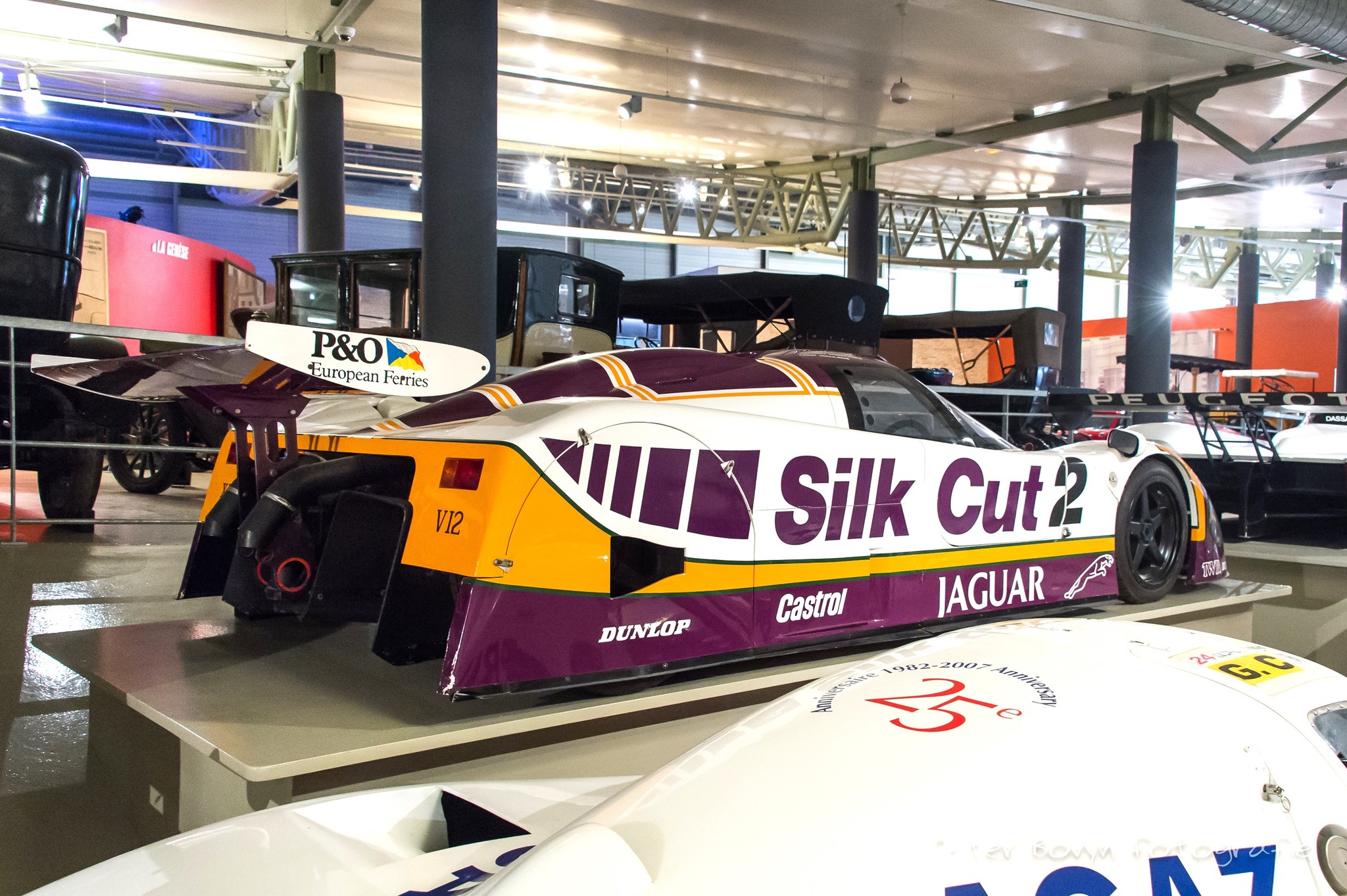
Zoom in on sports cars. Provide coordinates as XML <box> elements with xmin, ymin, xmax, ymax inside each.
<box><xmin>169</xmin><ymin>315</ymin><xmax>1227</xmax><ymax>700</ymax></box>
<box><xmin>33</xmin><ymin>612</ymin><xmax>1347</xmax><ymax>896</ymax></box>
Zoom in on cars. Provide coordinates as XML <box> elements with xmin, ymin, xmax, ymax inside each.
<box><xmin>0</xmin><ymin>125</ymin><xmax>1347</xmax><ymax>523</ymax></box>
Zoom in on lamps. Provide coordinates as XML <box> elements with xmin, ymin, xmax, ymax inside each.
<box><xmin>1313</xmin><ymin>208</ymin><xmax>1326</xmax><ymax>255</ymax></box>
<box><xmin>889</xmin><ymin>0</ymin><xmax>912</xmax><ymax>105</ymax></box>
<box><xmin>699</xmin><ymin>185</ymin><xmax>708</xmax><ymax>203</ymax></box>
<box><xmin>17</xmin><ymin>59</ymin><xmax>43</xmax><ymax>112</ymax></box>
<box><xmin>612</xmin><ymin>114</ymin><xmax>629</xmax><ymax>178</ymax></box>
<box><xmin>555</xmin><ymin>155</ymin><xmax>572</xmax><ymax>188</ymax></box>
<box><xmin>618</xmin><ymin>94</ymin><xmax>642</xmax><ymax>119</ymax></box>
<box><xmin>409</xmin><ymin>175</ymin><xmax>422</xmax><ymax>191</ymax></box>
<box><xmin>719</xmin><ymin>188</ymin><xmax>729</xmax><ymax>204</ymax></box>
<box><xmin>102</xmin><ymin>16</ymin><xmax>128</xmax><ymax>43</ymax></box>
